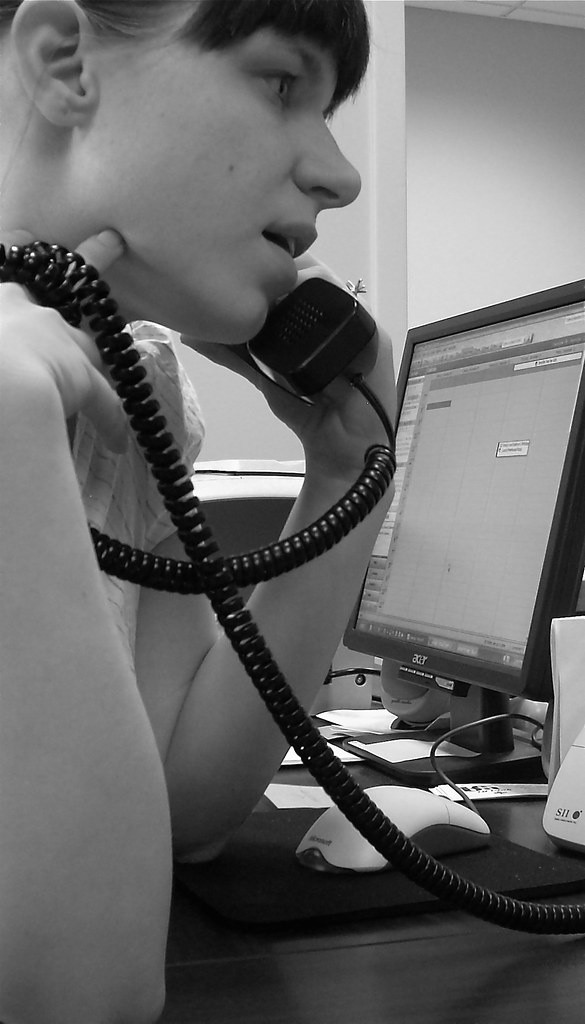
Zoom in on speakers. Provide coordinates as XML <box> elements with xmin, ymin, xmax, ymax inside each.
<box><xmin>379</xmin><ymin>658</ymin><xmax>454</xmax><ymax>731</ymax></box>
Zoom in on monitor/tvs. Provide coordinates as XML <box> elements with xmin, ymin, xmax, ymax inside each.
<box><xmin>341</xmin><ymin>278</ymin><xmax>585</xmax><ymax>788</ymax></box>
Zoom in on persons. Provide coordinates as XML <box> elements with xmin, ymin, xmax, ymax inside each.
<box><xmin>0</xmin><ymin>1</ymin><xmax>398</xmax><ymax>1024</ymax></box>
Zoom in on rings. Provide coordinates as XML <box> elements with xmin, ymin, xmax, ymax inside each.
<box><xmin>346</xmin><ymin>278</ymin><xmax>367</xmax><ymax>296</ymax></box>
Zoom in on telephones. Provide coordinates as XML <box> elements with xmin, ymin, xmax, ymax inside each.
<box><xmin>232</xmin><ymin>277</ymin><xmax>380</xmax><ymax>408</ymax></box>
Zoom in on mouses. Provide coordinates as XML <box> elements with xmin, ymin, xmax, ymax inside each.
<box><xmin>295</xmin><ymin>785</ymin><xmax>494</xmax><ymax>876</ymax></box>
